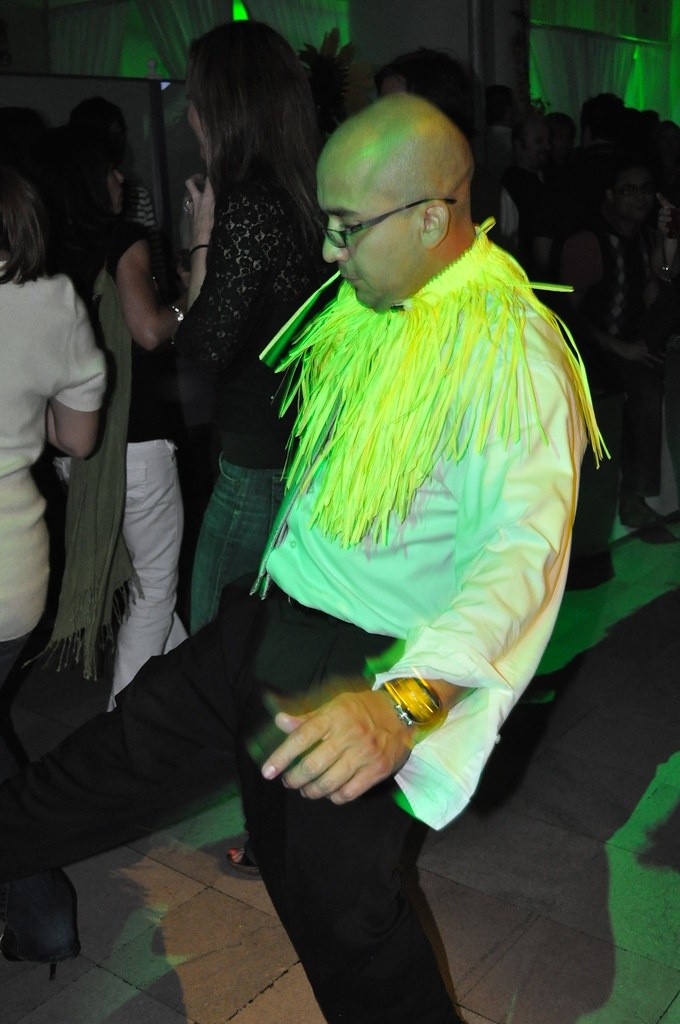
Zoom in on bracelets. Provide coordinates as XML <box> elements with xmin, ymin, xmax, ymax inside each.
<box><xmin>189</xmin><ymin>244</ymin><xmax>208</xmax><ymax>257</ymax></box>
<box><xmin>167</xmin><ymin>303</ymin><xmax>186</xmax><ymax>327</ymax></box>
<box><xmin>382</xmin><ymin>669</ymin><xmax>442</xmax><ymax>730</ymax></box>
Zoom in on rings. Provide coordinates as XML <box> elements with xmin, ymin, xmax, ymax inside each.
<box><xmin>647</xmin><ymin>353</ymin><xmax>650</xmax><ymax>359</ymax></box>
<box><xmin>183</xmin><ymin>200</ymin><xmax>192</xmax><ymax>207</ymax></box>
<box><xmin>182</xmin><ymin>206</ymin><xmax>188</xmax><ymax>213</ymax></box>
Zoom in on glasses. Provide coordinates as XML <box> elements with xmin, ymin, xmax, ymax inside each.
<box><xmin>311</xmin><ymin>198</ymin><xmax>457</xmax><ymax>248</ymax></box>
<box><xmin>618</xmin><ymin>181</ymin><xmax>660</xmax><ymax>196</ymax></box>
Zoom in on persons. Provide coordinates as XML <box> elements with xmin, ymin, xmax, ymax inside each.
<box><xmin>0</xmin><ymin>93</ymin><xmax>611</xmax><ymax>1024</ymax></box>
<box><xmin>0</xmin><ymin>19</ymin><xmax>680</xmax><ymax>960</ymax></box>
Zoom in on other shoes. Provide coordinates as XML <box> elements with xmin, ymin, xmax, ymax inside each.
<box><xmin>227</xmin><ymin>848</ymin><xmax>259</xmax><ymax>873</ymax></box>
<box><xmin>618</xmin><ymin>497</ymin><xmax>663</xmax><ymax>527</ymax></box>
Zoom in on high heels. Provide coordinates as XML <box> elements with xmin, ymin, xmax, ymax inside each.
<box><xmin>0</xmin><ymin>924</ymin><xmax>80</xmax><ymax>981</ymax></box>
